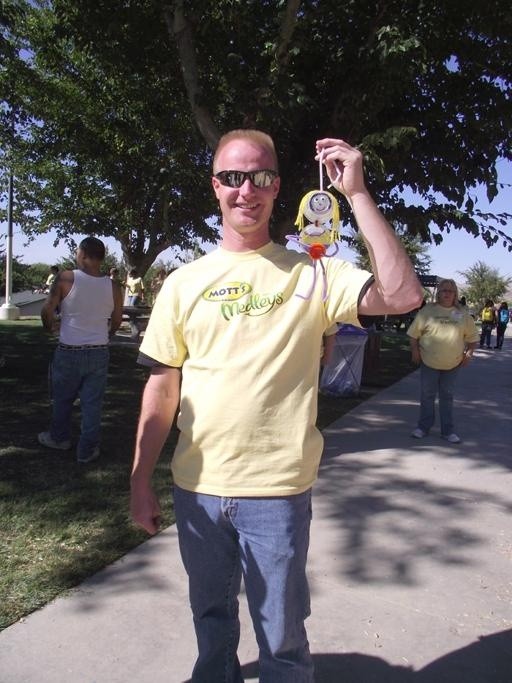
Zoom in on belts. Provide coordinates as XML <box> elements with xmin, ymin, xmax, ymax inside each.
<box><xmin>42</xmin><ymin>343</ymin><xmax>109</xmax><ymax>408</ymax></box>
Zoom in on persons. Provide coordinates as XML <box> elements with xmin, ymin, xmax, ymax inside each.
<box><xmin>38</xmin><ymin>237</ymin><xmax>125</xmax><ymax>462</ymax></box>
<box><xmin>458</xmin><ymin>296</ymin><xmax>468</xmax><ymax>313</ymax></box>
<box><xmin>46</xmin><ymin>264</ymin><xmax>60</xmax><ymax>289</ymax></box>
<box><xmin>476</xmin><ymin>298</ymin><xmax>499</xmax><ymax>349</ymax></box>
<box><xmin>125</xmin><ymin>268</ymin><xmax>146</xmax><ymax>306</ymax></box>
<box><xmin>125</xmin><ymin>126</ymin><xmax>430</xmax><ymax>681</ymax></box>
<box><xmin>109</xmin><ymin>267</ymin><xmax>125</xmax><ymax>285</ymax></box>
<box><xmin>493</xmin><ymin>301</ymin><xmax>509</xmax><ymax>349</ymax></box>
<box><xmin>405</xmin><ymin>277</ymin><xmax>481</xmax><ymax>442</ymax></box>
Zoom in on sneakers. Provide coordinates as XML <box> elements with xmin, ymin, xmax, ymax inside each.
<box><xmin>440</xmin><ymin>433</ymin><xmax>461</xmax><ymax>443</ymax></box>
<box><xmin>411</xmin><ymin>428</ymin><xmax>428</xmax><ymax>438</ymax></box>
<box><xmin>38</xmin><ymin>432</ymin><xmax>73</xmax><ymax>450</ymax></box>
<box><xmin>77</xmin><ymin>446</ymin><xmax>100</xmax><ymax>464</ymax></box>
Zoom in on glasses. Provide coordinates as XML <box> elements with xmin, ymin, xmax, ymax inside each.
<box><xmin>215</xmin><ymin>168</ymin><xmax>276</xmax><ymax>190</ymax></box>
